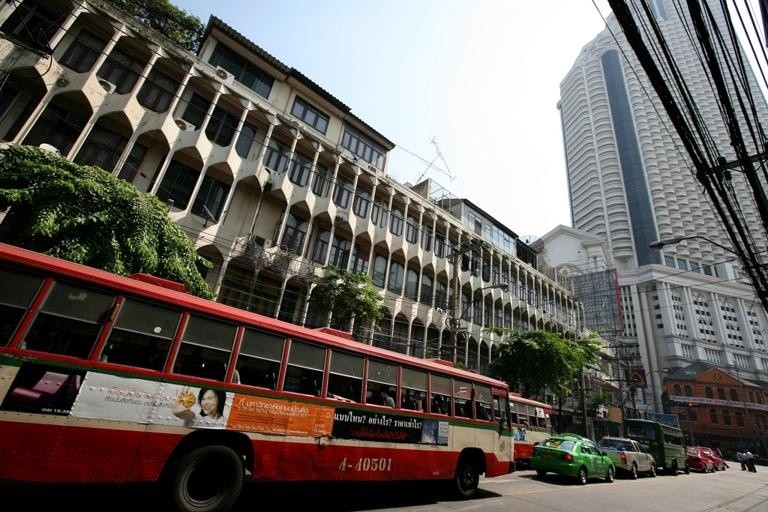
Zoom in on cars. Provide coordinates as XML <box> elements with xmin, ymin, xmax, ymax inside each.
<box><xmin>529</xmin><ymin>433</ymin><xmax>616</xmax><ymax>486</ymax></box>
<box><xmin>686</xmin><ymin>447</ymin><xmax>723</xmax><ymax>473</ymax></box>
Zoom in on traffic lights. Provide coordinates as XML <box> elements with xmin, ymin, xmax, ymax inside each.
<box><xmin>675</xmin><ymin>401</ymin><xmax>701</xmax><ymax>407</ymax></box>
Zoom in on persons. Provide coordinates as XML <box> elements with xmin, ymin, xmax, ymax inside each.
<box><xmin>736</xmin><ymin>447</ymin><xmax>757</xmax><ymax>473</ymax></box>
<box><xmin>173</xmin><ymin>386</ymin><xmax>227</xmax><ymax>429</ymax></box>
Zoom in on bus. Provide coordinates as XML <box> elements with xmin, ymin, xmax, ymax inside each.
<box><xmin>506</xmin><ymin>392</ymin><xmax>554</xmax><ymax>470</ymax></box>
<box><xmin>0</xmin><ymin>241</ymin><xmax>518</xmax><ymax>512</ymax></box>
<box><xmin>625</xmin><ymin>419</ymin><xmax>687</xmax><ymax>476</ymax></box>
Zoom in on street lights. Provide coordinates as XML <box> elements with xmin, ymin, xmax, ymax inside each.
<box><xmin>649</xmin><ymin>235</ymin><xmax>768</xmax><ymax>271</ymax></box>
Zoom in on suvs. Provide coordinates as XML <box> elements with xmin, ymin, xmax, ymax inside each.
<box><xmin>597</xmin><ymin>436</ymin><xmax>657</xmax><ymax>479</ymax></box>
